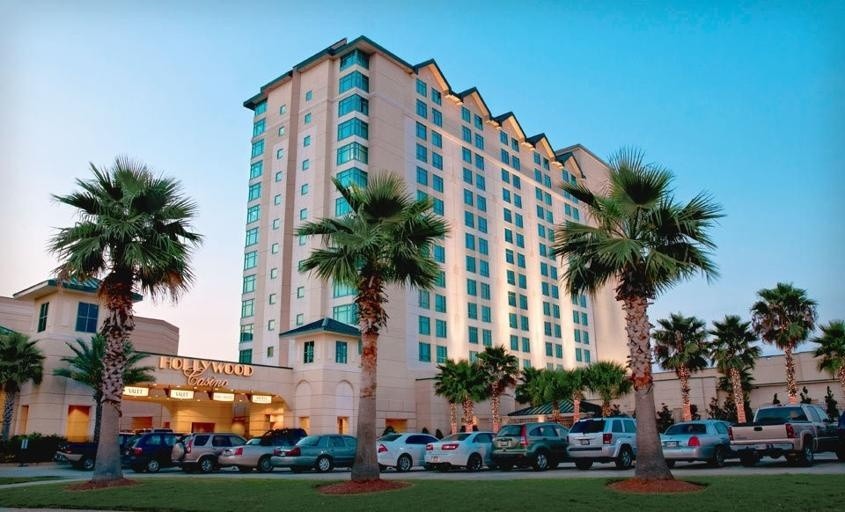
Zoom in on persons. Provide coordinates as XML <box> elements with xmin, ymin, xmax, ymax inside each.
<box><xmin>382</xmin><ymin>426</ymin><xmax>396</xmax><ymax>435</ymax></box>
<box><xmin>422</xmin><ymin>427</ymin><xmax>443</xmax><ymax>440</ymax></box>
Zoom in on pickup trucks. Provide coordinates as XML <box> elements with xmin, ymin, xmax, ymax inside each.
<box><xmin>728</xmin><ymin>402</ymin><xmax>844</xmax><ymax>467</ymax></box>
